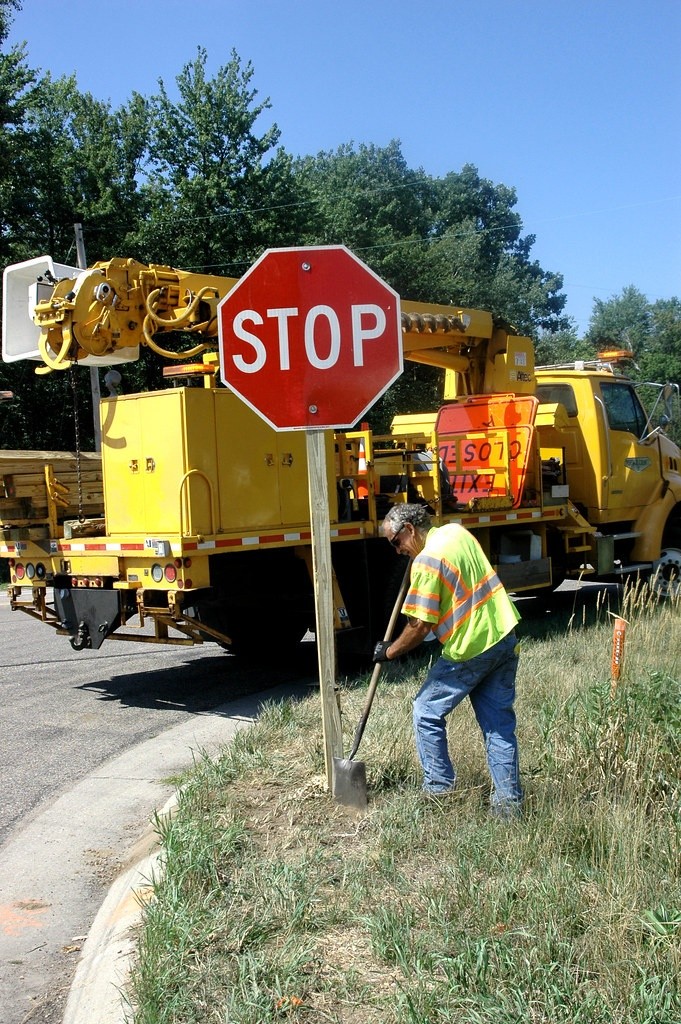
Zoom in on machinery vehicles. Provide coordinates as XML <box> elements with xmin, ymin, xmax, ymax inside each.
<box><xmin>1</xmin><ymin>258</ymin><xmax>681</xmax><ymax>676</ymax></box>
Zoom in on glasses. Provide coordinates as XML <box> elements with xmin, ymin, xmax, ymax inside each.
<box><xmin>390</xmin><ymin>523</ymin><xmax>404</xmax><ymax>548</ymax></box>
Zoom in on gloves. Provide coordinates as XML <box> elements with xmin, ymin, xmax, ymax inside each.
<box><xmin>372</xmin><ymin>640</ymin><xmax>393</xmax><ymax>662</ymax></box>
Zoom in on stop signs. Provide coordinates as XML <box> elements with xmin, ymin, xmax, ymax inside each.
<box><xmin>216</xmin><ymin>245</ymin><xmax>405</xmax><ymax>432</ymax></box>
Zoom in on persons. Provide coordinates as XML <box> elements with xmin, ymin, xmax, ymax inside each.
<box><xmin>372</xmin><ymin>505</ymin><xmax>523</xmax><ymax>819</ymax></box>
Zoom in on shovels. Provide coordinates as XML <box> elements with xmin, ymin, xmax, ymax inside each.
<box><xmin>332</xmin><ymin>558</ymin><xmax>415</xmax><ymax>807</ymax></box>
